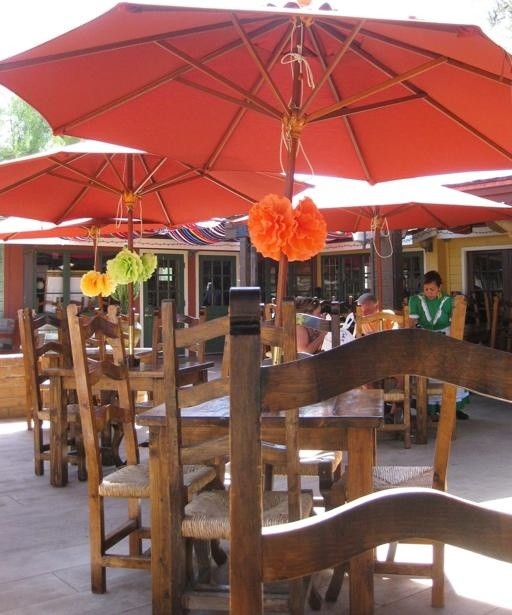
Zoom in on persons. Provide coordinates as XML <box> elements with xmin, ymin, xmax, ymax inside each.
<box><xmin>290</xmin><ymin>295</ymin><xmax>333</xmax><ymax>356</ymax></box>
<box><xmin>405</xmin><ymin>270</ymin><xmax>470</xmax><ymax>421</ymax></box>
<box><xmin>352</xmin><ymin>291</ymin><xmax>405</xmax><ymax>425</ymax></box>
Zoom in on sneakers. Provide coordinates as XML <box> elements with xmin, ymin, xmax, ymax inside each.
<box><xmin>457</xmin><ymin>411</ymin><xmax>470</xmax><ymax>420</ymax></box>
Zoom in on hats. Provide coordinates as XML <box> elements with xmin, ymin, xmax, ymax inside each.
<box><xmin>354</xmin><ymin>293</ymin><xmax>378</xmax><ymax>304</ymax></box>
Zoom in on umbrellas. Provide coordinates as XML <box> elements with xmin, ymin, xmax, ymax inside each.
<box><xmin>0</xmin><ymin>138</ymin><xmax>321</xmax><ymax>366</ymax></box>
<box><xmin>0</xmin><ymin>211</ymin><xmax>231</xmax><ymax>367</ymax></box>
<box><xmin>227</xmin><ymin>173</ymin><xmax>511</xmax><ymax>310</ymax></box>
<box><xmin>0</xmin><ymin>3</ymin><xmax>512</xmax><ymax>365</ymax></box>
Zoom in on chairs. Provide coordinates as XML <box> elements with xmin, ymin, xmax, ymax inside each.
<box><xmin>17</xmin><ymin>284</ymin><xmax>512</xmax><ymax>615</ymax></box>
